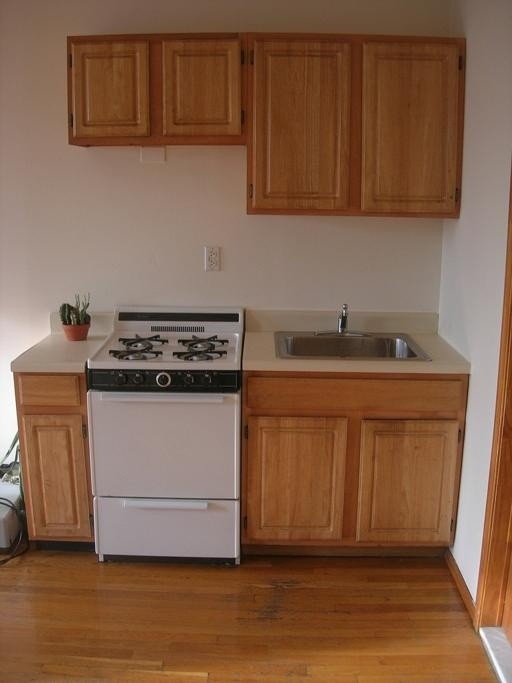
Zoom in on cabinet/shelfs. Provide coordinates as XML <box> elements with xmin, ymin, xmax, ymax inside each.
<box><xmin>67</xmin><ymin>32</ymin><xmax>248</xmax><ymax>147</ymax></box>
<box><xmin>13</xmin><ymin>374</ymin><xmax>94</xmax><ymax>551</ymax></box>
<box><xmin>241</xmin><ymin>372</ymin><xmax>470</xmax><ymax>558</ymax></box>
<box><xmin>246</xmin><ymin>32</ymin><xmax>466</xmax><ymax>219</ymax></box>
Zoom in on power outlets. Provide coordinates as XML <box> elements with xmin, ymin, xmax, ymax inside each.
<box><xmin>205</xmin><ymin>247</ymin><xmax>220</xmax><ymax>272</ymax></box>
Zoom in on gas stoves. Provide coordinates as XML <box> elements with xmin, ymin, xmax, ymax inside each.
<box><xmin>84</xmin><ymin>330</ymin><xmax>242</xmax><ymax>393</ymax></box>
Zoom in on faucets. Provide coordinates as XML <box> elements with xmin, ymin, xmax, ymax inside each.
<box><xmin>338</xmin><ymin>304</ymin><xmax>347</xmax><ymax>334</ymax></box>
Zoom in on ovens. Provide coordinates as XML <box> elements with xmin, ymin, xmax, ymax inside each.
<box><xmin>84</xmin><ymin>395</ymin><xmax>240</xmax><ymax>564</ymax></box>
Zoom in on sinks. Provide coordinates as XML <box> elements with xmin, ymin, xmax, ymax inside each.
<box><xmin>274</xmin><ymin>330</ymin><xmax>430</xmax><ymax>360</ymax></box>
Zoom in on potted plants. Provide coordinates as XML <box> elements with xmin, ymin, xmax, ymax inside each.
<box><xmin>58</xmin><ymin>291</ymin><xmax>91</xmax><ymax>341</ymax></box>
